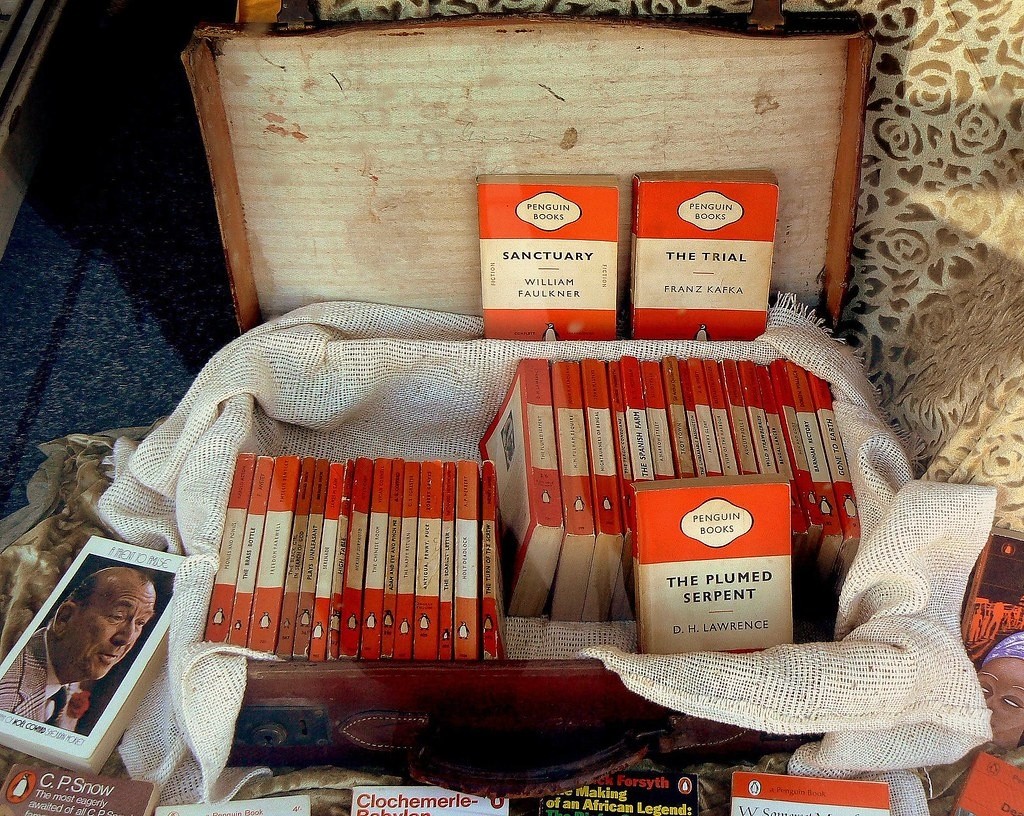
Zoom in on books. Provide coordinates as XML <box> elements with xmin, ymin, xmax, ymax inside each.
<box><xmin>476</xmin><ymin>174</ymin><xmax>619</xmax><ymax>341</ymax></box>
<box><xmin>202</xmin><ymin>452</ymin><xmax>507</xmax><ymax>661</ymax></box>
<box><xmin>949</xmin><ymin>526</ymin><xmax>1024</xmax><ymax>816</ymax></box>
<box><xmin>0</xmin><ymin>535</ymin><xmax>188</xmax><ymax>776</ymax></box>
<box><xmin>628</xmin><ymin>167</ymin><xmax>779</xmax><ymax>340</ymax></box>
<box><xmin>479</xmin><ymin>355</ymin><xmax>860</xmax><ymax>655</ymax></box>
<box><xmin>0</xmin><ymin>764</ymin><xmax>890</xmax><ymax>816</ymax></box>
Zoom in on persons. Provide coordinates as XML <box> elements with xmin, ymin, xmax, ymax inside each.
<box><xmin>0</xmin><ymin>567</ymin><xmax>156</xmax><ymax>732</ymax></box>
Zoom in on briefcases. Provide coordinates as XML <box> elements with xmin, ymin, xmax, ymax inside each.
<box><xmin>169</xmin><ymin>0</ymin><xmax>871</xmax><ymax>775</ymax></box>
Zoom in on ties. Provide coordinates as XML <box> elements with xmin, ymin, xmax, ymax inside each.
<box><xmin>45</xmin><ymin>689</ymin><xmax>67</xmax><ymax>725</ymax></box>
